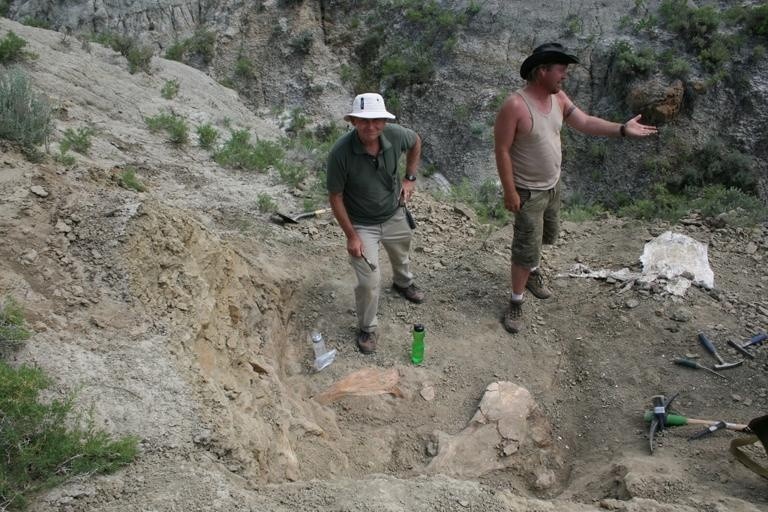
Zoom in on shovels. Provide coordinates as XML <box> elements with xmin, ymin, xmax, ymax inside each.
<box><xmin>277</xmin><ymin>207</ymin><xmax>331</xmax><ymax>224</ymax></box>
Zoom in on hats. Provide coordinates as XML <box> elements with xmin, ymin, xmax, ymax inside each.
<box><xmin>343</xmin><ymin>93</ymin><xmax>396</xmax><ymax>122</ymax></box>
<box><xmin>520</xmin><ymin>42</ymin><xmax>581</xmax><ymax>80</ymax></box>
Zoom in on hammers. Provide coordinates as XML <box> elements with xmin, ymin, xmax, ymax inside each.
<box><xmin>728</xmin><ymin>334</ymin><xmax>768</xmax><ymax>359</ymax></box>
<box><xmin>698</xmin><ymin>333</ymin><xmax>745</xmax><ymax>370</ymax></box>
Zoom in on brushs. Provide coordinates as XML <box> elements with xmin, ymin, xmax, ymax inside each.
<box><xmin>362</xmin><ymin>252</ymin><xmax>375</xmax><ymax>271</ymax></box>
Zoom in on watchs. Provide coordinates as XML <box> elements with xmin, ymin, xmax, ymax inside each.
<box><xmin>404</xmin><ymin>175</ymin><xmax>416</xmax><ymax>181</ymax></box>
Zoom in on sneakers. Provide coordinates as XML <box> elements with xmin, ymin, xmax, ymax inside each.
<box><xmin>357</xmin><ymin>329</ymin><xmax>377</xmax><ymax>354</ymax></box>
<box><xmin>504</xmin><ymin>293</ymin><xmax>526</xmax><ymax>333</ymax></box>
<box><xmin>526</xmin><ymin>270</ymin><xmax>551</xmax><ymax>298</ymax></box>
<box><xmin>391</xmin><ymin>282</ymin><xmax>424</xmax><ymax>303</ymax></box>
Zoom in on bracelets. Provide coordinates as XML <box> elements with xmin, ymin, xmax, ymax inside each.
<box><xmin>621</xmin><ymin>125</ymin><xmax>625</xmax><ymax>137</ymax></box>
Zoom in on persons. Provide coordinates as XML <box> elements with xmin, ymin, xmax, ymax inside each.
<box><xmin>327</xmin><ymin>92</ymin><xmax>424</xmax><ymax>353</ymax></box>
<box><xmin>495</xmin><ymin>42</ymin><xmax>658</xmax><ymax>332</ymax></box>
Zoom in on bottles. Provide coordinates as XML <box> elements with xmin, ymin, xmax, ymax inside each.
<box><xmin>411</xmin><ymin>323</ymin><xmax>426</xmax><ymax>365</ymax></box>
<box><xmin>311</xmin><ymin>334</ymin><xmax>327</xmax><ymax>357</ymax></box>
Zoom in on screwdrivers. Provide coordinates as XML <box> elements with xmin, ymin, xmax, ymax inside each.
<box><xmin>675</xmin><ymin>359</ymin><xmax>726</xmax><ymax>379</ymax></box>
